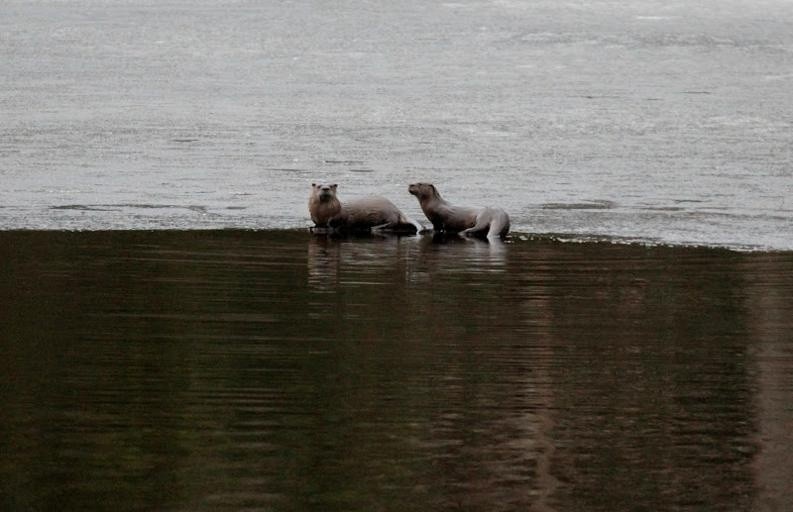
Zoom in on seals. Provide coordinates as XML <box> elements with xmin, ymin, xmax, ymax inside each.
<box><xmin>407</xmin><ymin>182</ymin><xmax>510</xmax><ymax>245</ymax></box>
<box><xmin>307</xmin><ymin>181</ymin><xmax>417</xmax><ymax>237</ymax></box>
